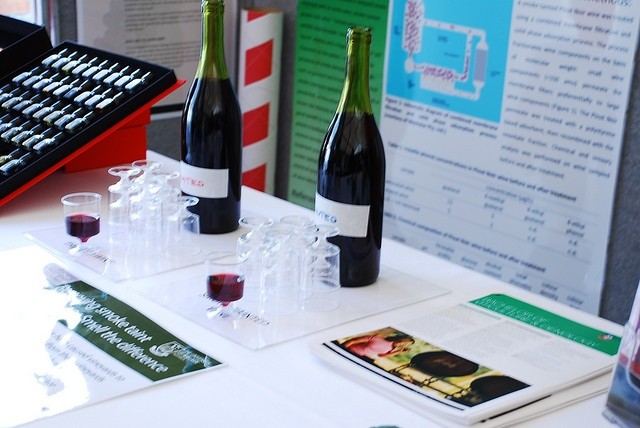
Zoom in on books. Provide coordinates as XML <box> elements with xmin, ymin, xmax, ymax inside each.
<box><xmin>312</xmin><ymin>289</ymin><xmax>626</xmax><ymax>426</ymax></box>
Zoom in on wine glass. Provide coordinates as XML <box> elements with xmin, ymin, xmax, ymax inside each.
<box><xmin>236</xmin><ymin>214</ymin><xmax>342</xmax><ymax>317</ymax></box>
<box><xmin>107</xmin><ymin>159</ymin><xmax>202</xmax><ymax>261</ymax></box>
<box><xmin>60</xmin><ymin>192</ymin><xmax>103</xmax><ymax>257</ymax></box>
<box><xmin>203</xmin><ymin>249</ymin><xmax>248</xmax><ymax>323</ymax></box>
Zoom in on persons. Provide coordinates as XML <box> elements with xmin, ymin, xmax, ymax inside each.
<box><xmin>340</xmin><ymin>334</ymin><xmax>415</xmax><ymax>361</ymax></box>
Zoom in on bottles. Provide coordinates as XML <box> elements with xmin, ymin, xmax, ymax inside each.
<box><xmin>313</xmin><ymin>25</ymin><xmax>387</xmax><ymax>289</ymax></box>
<box><xmin>178</xmin><ymin>0</ymin><xmax>243</xmax><ymax>235</ymax></box>
